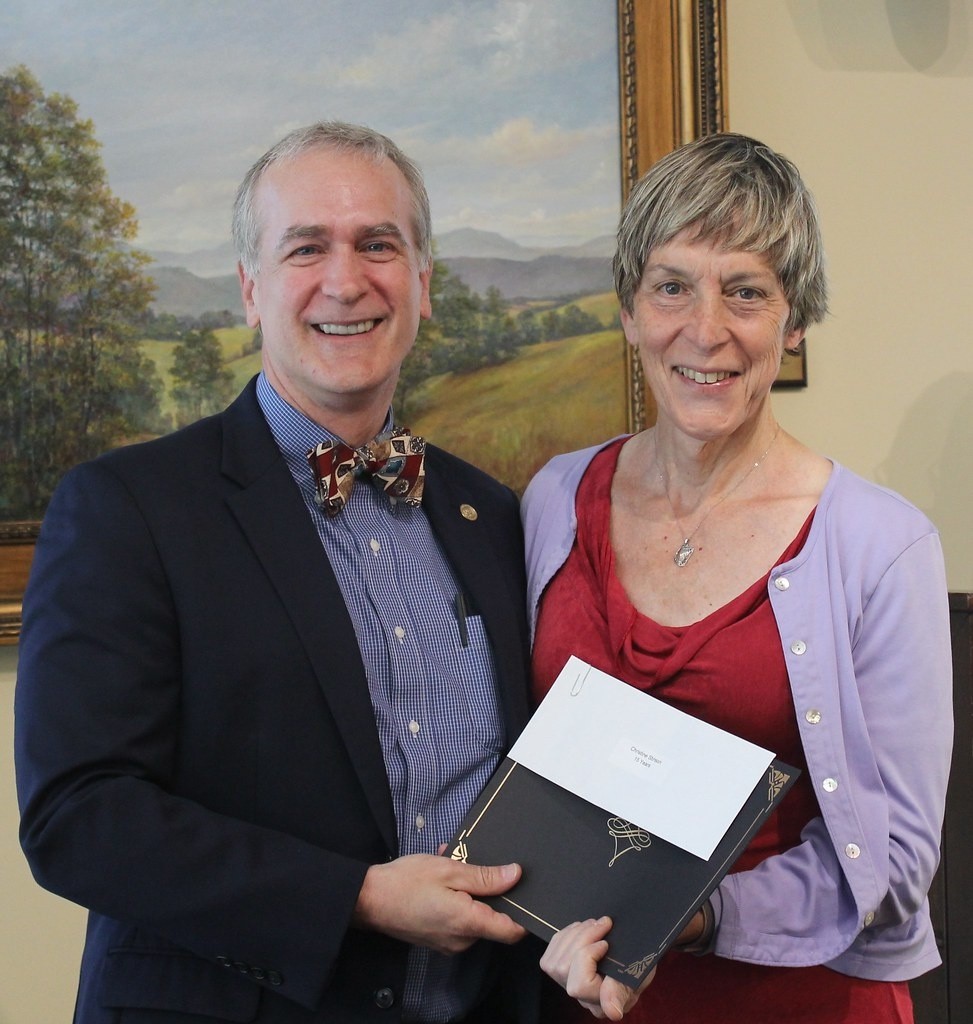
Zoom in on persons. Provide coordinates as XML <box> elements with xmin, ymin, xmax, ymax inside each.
<box><xmin>520</xmin><ymin>134</ymin><xmax>954</xmax><ymax>1024</ymax></box>
<box><xmin>12</xmin><ymin>124</ymin><xmax>531</xmax><ymax>1024</ymax></box>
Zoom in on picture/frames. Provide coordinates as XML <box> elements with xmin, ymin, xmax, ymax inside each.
<box><xmin>0</xmin><ymin>0</ymin><xmax>729</xmax><ymax>648</ymax></box>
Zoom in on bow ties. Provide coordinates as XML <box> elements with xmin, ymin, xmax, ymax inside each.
<box><xmin>306</xmin><ymin>430</ymin><xmax>428</xmax><ymax>519</ymax></box>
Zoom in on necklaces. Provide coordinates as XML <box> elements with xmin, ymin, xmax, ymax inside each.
<box><xmin>655</xmin><ymin>421</ymin><xmax>780</xmax><ymax>566</ymax></box>
